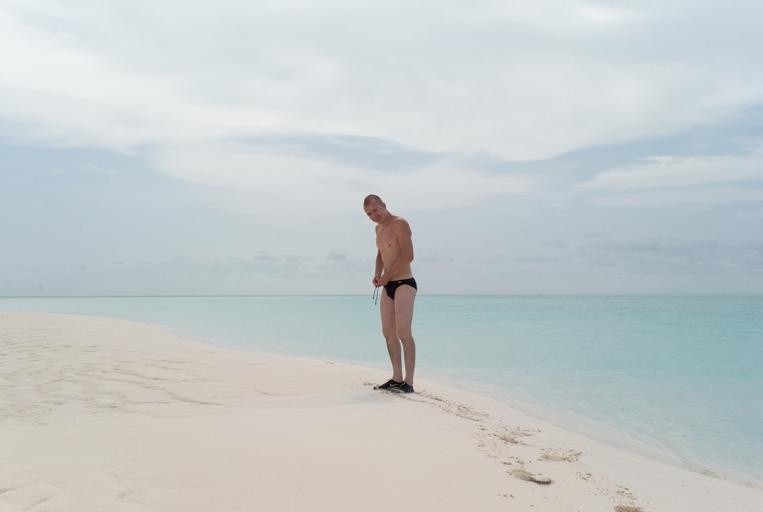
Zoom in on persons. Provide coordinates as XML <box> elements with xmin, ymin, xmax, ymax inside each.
<box><xmin>363</xmin><ymin>195</ymin><xmax>418</xmax><ymax>395</ymax></box>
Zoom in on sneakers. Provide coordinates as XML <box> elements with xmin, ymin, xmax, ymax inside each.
<box><xmin>391</xmin><ymin>382</ymin><xmax>413</xmax><ymax>393</ymax></box>
<box><xmin>374</xmin><ymin>379</ymin><xmax>405</xmax><ymax>390</ymax></box>
